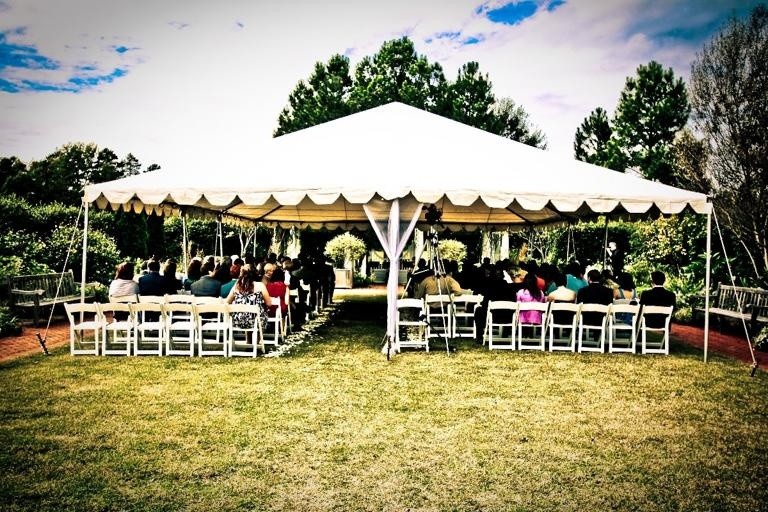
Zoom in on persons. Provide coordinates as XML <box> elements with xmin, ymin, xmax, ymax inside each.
<box><xmin>109</xmin><ymin>253</ymin><xmax>335</xmax><ymax>352</ymax></box>
<box><xmin>405</xmin><ymin>257</ymin><xmax>677</xmax><ymax>345</ymax></box>
<box><xmin>606</xmin><ymin>241</ymin><xmax>623</xmax><ymax>272</ymax></box>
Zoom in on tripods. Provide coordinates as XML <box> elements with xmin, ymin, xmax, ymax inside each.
<box><xmin>377</xmin><ymin>224</ymin><xmax>464</xmax><ymax>358</ymax></box>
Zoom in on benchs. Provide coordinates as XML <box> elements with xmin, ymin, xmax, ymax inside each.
<box><xmin>692</xmin><ymin>282</ymin><xmax>768</xmax><ymax>341</ymax></box>
<box><xmin>7</xmin><ymin>268</ymin><xmax>99</xmax><ymax>328</ymax></box>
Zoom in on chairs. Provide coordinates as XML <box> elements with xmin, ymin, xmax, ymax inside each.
<box><xmin>63</xmin><ymin>282</ymin><xmax>331</xmax><ymax>358</ymax></box>
<box><xmin>395</xmin><ymin>293</ymin><xmax>674</xmax><ymax>356</ymax></box>
